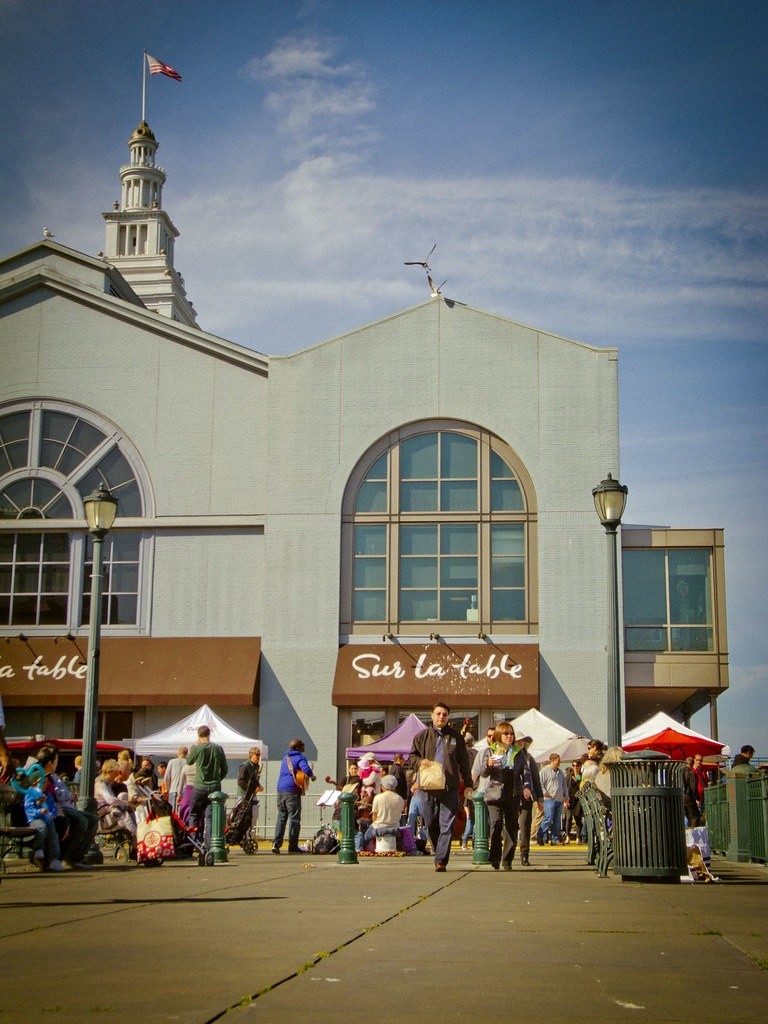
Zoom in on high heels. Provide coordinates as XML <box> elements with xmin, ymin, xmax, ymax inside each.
<box><xmin>565</xmin><ymin>836</ymin><xmax>583</xmax><ymax>845</ymax></box>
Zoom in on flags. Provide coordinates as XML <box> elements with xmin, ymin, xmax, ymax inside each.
<box><xmin>146</xmin><ymin>54</ymin><xmax>183</xmax><ymax>81</ymax></box>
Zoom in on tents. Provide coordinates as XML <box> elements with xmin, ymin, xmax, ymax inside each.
<box><xmin>345</xmin><ymin>714</ymin><xmax>429</xmax><ymax>774</ymax></box>
<box><xmin>132</xmin><ymin>704</ymin><xmax>268</xmax><ymax>841</ymax></box>
<box><xmin>473</xmin><ymin>708</ymin><xmax>594</xmax><ymax>763</ymax></box>
<box><xmin>622</xmin><ymin>712</ymin><xmax>730</xmax><ymax>771</ymax></box>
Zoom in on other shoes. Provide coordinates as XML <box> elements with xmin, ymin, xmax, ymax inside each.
<box><xmin>73</xmin><ymin>860</ymin><xmax>92</xmax><ymax>870</ymax></box>
<box><xmin>272</xmin><ymin>841</ymin><xmax>281</xmax><ymax>854</ymax></box>
<box><xmin>521</xmin><ymin>857</ymin><xmax>531</xmax><ymax>866</ymax></box>
<box><xmin>288</xmin><ymin>847</ymin><xmax>306</xmax><ymax>854</ymax></box>
<box><xmin>406</xmin><ymin>850</ymin><xmax>425</xmax><ymax>856</ymax></box>
<box><xmin>491</xmin><ymin>863</ymin><xmax>512</xmax><ymax>870</ymax></box>
<box><xmin>48</xmin><ymin>858</ymin><xmax>64</xmax><ymax>872</ymax></box>
<box><xmin>35</xmin><ymin>849</ymin><xmax>44</xmax><ymax>858</ymax></box>
<box><xmin>537</xmin><ymin>836</ymin><xmax>564</xmax><ymax>846</ymax></box>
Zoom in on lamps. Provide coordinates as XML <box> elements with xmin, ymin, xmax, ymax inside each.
<box><xmin>356</xmin><ymin>719</ymin><xmax>365</xmax><ymax>734</ymax></box>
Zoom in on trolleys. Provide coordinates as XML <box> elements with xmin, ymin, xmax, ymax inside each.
<box><xmin>224</xmin><ymin>775</ymin><xmax>259</xmax><ymax>854</ymax></box>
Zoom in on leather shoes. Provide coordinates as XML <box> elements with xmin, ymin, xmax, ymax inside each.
<box><xmin>433</xmin><ymin>860</ymin><xmax>446</xmax><ymax>871</ymax></box>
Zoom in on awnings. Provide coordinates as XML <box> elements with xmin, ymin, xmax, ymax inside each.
<box><xmin>0</xmin><ymin>636</ymin><xmax>261</xmax><ymax>710</ymax></box>
<box><xmin>331</xmin><ymin>642</ymin><xmax>540</xmax><ymax>709</ymax></box>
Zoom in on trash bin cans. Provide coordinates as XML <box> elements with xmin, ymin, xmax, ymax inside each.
<box><xmin>604</xmin><ymin>750</ymin><xmax>690</xmax><ymax>884</ymax></box>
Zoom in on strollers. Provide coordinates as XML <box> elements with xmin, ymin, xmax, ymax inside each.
<box><xmin>133</xmin><ymin>777</ymin><xmax>213</xmax><ymax>866</ymax></box>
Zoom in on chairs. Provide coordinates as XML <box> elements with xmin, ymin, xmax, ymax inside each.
<box><xmin>581</xmin><ymin>781</ymin><xmax>614</xmax><ymax>878</ymax></box>
<box><xmin>575</xmin><ymin>790</ymin><xmax>600</xmax><ymax>865</ymax></box>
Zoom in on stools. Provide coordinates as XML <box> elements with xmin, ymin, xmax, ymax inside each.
<box><xmin>375</xmin><ymin>828</ymin><xmax>399</xmax><ymax>852</ymax></box>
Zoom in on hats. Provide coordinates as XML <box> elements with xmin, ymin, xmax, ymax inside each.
<box><xmin>381</xmin><ymin>775</ymin><xmax>398</xmax><ymax>790</ymax></box>
<box><xmin>342</xmin><ymin>782</ymin><xmax>359</xmax><ymax>797</ymax></box>
<box><xmin>359</xmin><ymin>752</ymin><xmax>375</xmax><ymax>760</ymax></box>
<box><xmin>358</xmin><ymin>760</ymin><xmax>369</xmax><ymax>770</ymax></box>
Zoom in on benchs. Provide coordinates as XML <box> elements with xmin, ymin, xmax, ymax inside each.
<box><xmin>0</xmin><ymin>792</ymin><xmax>40</xmax><ymax>873</ymax></box>
<box><xmin>95</xmin><ymin>804</ymin><xmax>135</xmax><ymax>863</ymax></box>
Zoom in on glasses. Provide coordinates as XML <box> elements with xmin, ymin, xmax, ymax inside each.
<box><xmin>587</xmin><ymin>747</ymin><xmax>591</xmax><ymax>750</ymax></box>
<box><xmin>486</xmin><ymin>734</ymin><xmax>493</xmax><ymax>737</ymax></box>
<box><xmin>255</xmin><ymin>753</ymin><xmax>261</xmax><ymax>757</ymax></box>
<box><xmin>433</xmin><ymin>711</ymin><xmax>451</xmax><ymax>717</ymax></box>
<box><xmin>694</xmin><ymin>758</ymin><xmax>704</xmax><ymax>762</ymax></box>
<box><xmin>504</xmin><ymin>732</ymin><xmax>514</xmax><ymax>736</ymax></box>
<box><xmin>572</xmin><ymin>764</ymin><xmax>580</xmax><ymax>767</ymax></box>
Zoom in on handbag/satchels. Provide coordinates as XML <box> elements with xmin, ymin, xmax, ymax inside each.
<box><xmin>485</xmin><ymin>782</ymin><xmax>505</xmax><ymax>804</ymax></box>
<box><xmin>136</xmin><ymin>810</ymin><xmax>176</xmax><ymax>864</ymax></box>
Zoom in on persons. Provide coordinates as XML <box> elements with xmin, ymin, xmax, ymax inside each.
<box><xmin>333</xmin><ymin>703</ymin><xmax>626</xmax><ymax>873</ymax></box>
<box><xmin>0</xmin><ymin>708</ymin><xmax>264</xmax><ymax>874</ymax></box>
<box><xmin>678</xmin><ymin>746</ymin><xmax>755</xmax><ymax>826</ymax></box>
<box><xmin>271</xmin><ymin>739</ymin><xmax>316</xmax><ymax>854</ymax></box>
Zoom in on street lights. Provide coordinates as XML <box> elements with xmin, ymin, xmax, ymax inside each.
<box><xmin>591</xmin><ymin>471</ymin><xmax>628</xmax><ymax>750</ymax></box>
<box><xmin>76</xmin><ymin>483</ymin><xmax>120</xmax><ymax>807</ymax></box>
